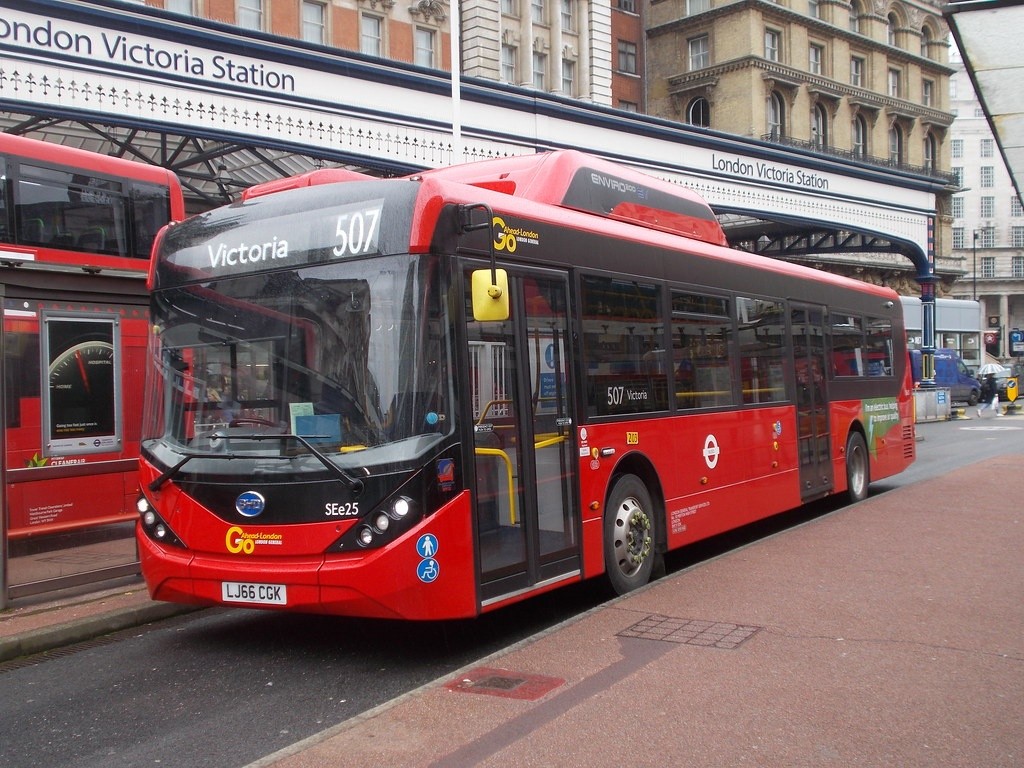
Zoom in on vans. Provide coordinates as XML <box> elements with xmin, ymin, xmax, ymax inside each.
<box><xmin>908</xmin><ymin>348</ymin><xmax>982</xmax><ymax>407</ymax></box>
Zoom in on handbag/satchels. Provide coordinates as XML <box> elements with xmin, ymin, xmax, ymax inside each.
<box><xmin>991</xmin><ymin>396</ymin><xmax>999</xmax><ymax>410</ymax></box>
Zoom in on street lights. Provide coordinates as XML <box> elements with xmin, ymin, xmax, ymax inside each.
<box><xmin>972</xmin><ymin>228</ymin><xmax>986</xmax><ymax>301</ymax></box>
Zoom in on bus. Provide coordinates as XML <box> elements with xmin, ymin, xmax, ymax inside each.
<box><xmin>132</xmin><ymin>150</ymin><xmax>924</xmax><ymax>620</ymax></box>
<box><xmin>0</xmin><ymin>130</ymin><xmax>187</xmax><ymax>544</ymax></box>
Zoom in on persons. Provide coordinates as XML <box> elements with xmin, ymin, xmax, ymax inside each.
<box><xmin>194</xmin><ymin>374</ymin><xmax>242</xmax><ymax>429</ymax></box>
<box><xmin>318</xmin><ymin>290</ymin><xmax>439</xmax><ymax>446</ymax></box>
<box><xmin>976</xmin><ymin>373</ymin><xmax>1005</xmax><ymax>418</ymax></box>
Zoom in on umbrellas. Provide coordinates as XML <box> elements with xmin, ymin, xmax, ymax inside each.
<box><xmin>976</xmin><ymin>363</ymin><xmax>1006</xmax><ymax>375</ymax></box>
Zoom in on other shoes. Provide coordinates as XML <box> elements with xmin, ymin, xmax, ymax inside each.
<box><xmin>977</xmin><ymin>408</ymin><xmax>983</xmax><ymax>417</ymax></box>
<box><xmin>997</xmin><ymin>413</ymin><xmax>1004</xmax><ymax>417</ymax></box>
